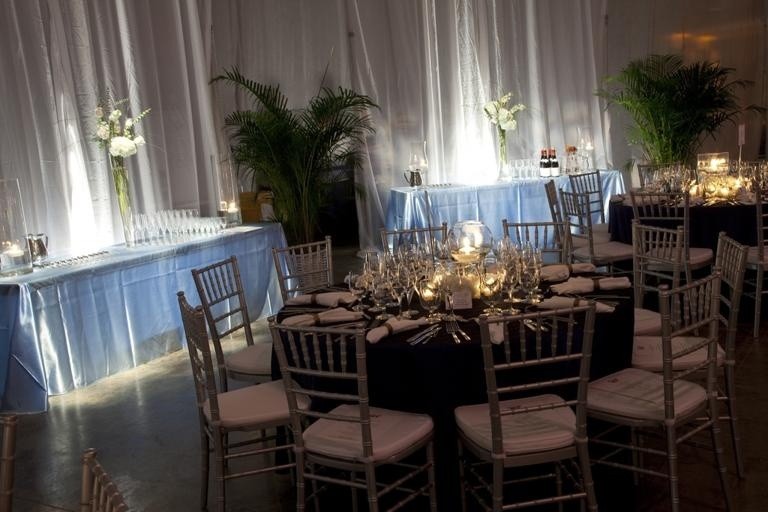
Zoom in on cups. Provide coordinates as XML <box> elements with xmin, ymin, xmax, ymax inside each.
<box><xmin>1</xmin><ymin>177</ymin><xmax>32</xmax><ymax>277</ymax></box>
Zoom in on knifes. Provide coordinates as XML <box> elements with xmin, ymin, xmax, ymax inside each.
<box><xmin>406</xmin><ymin>323</ymin><xmax>443</xmax><ymax>347</ymax></box>
<box><xmin>520</xmin><ymin>318</ymin><xmax>549</xmax><ymax>333</ymax></box>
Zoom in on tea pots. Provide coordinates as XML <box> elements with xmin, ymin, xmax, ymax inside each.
<box><xmin>24</xmin><ymin>233</ymin><xmax>50</xmax><ymax>262</ymax></box>
<box><xmin>403</xmin><ymin>168</ymin><xmax>422</xmax><ymax>188</ymax></box>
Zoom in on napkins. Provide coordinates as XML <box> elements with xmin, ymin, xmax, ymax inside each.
<box><xmin>539</xmin><ymin>260</ymin><xmax>597</xmax><ymax>281</ymax></box>
<box><xmin>285</xmin><ymin>290</ymin><xmax>359</xmax><ymax>307</ymax></box>
<box><xmin>366</xmin><ymin>314</ymin><xmax>428</xmax><ymax>344</ymax></box>
<box><xmin>538</xmin><ymin>295</ymin><xmax>615</xmax><ymax>313</ymax></box>
<box><xmin>281</xmin><ymin>308</ymin><xmax>363</xmax><ymax>327</ymax></box>
<box><xmin>551</xmin><ymin>274</ymin><xmax>632</xmax><ymax>295</ymax></box>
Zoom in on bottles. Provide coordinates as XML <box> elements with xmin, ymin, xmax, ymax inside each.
<box><xmin>539</xmin><ymin>147</ymin><xmax>560</xmax><ymax>178</ymax></box>
<box><xmin>576</xmin><ymin>127</ymin><xmax>593</xmax><ymax>173</ymax></box>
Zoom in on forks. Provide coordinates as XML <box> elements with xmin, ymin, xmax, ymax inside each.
<box><xmin>445</xmin><ymin>321</ymin><xmax>472</xmax><ymax>345</ymax></box>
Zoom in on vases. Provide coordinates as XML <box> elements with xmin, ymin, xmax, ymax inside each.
<box><xmin>106</xmin><ymin>152</ymin><xmax>140</xmax><ymax>249</ymax></box>
<box><xmin>494</xmin><ymin>124</ymin><xmax>511</xmax><ymax>183</ymax></box>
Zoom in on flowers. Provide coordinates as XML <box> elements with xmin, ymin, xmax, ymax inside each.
<box><xmin>80</xmin><ymin>83</ymin><xmax>170</xmax><ymax>218</ymax></box>
<box><xmin>480</xmin><ymin>91</ymin><xmax>545</xmax><ymax>163</ymax></box>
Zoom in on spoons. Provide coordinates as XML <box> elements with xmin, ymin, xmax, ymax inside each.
<box><xmin>326</xmin><ymin>319</ymin><xmax>364</xmax><ymax>330</ymax></box>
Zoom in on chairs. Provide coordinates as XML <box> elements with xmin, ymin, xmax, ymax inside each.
<box><xmin>175</xmin><ymin>291</ymin><xmax>310</xmax><ymax>508</ymax></box>
<box><xmin>578</xmin><ymin>267</ymin><xmax>736</xmax><ymax>510</ymax></box>
<box><xmin>543</xmin><ymin>180</ymin><xmax>605</xmax><ymax>261</ymax></box>
<box><xmin>568</xmin><ymin>170</ymin><xmax>610</xmax><ymax>237</ymax></box>
<box><xmin>734</xmin><ymin>180</ymin><xmax>765</xmax><ymax>339</ymax></box>
<box><xmin>380</xmin><ymin>223</ymin><xmax>448</xmax><ymax>265</ymax></box>
<box><xmin>0</xmin><ymin>412</ymin><xmax>20</xmax><ymax>511</ymax></box>
<box><xmin>557</xmin><ymin>188</ymin><xmax>634</xmax><ymax>285</ymax></box>
<box><xmin>731</xmin><ymin>160</ymin><xmax>764</xmax><ymax>180</ymax></box>
<box><xmin>456</xmin><ymin>297</ymin><xmax>598</xmax><ymax>509</ymax></box>
<box><xmin>77</xmin><ymin>446</ymin><xmax>133</xmax><ymax>511</ymax></box>
<box><xmin>629</xmin><ymin>190</ymin><xmax>714</xmax><ymax>308</ymax></box>
<box><xmin>623</xmin><ymin>220</ymin><xmax>681</xmax><ymax>334</ymax></box>
<box><xmin>188</xmin><ymin>252</ymin><xmax>284</xmax><ymax>442</ymax></box>
<box><xmin>630</xmin><ymin>232</ymin><xmax>749</xmax><ymax>482</ymax></box>
<box><xmin>269</xmin><ymin>234</ymin><xmax>336</xmax><ymax>301</ymax></box>
<box><xmin>637</xmin><ymin>161</ymin><xmax>692</xmax><ymax>185</ymax></box>
<box><xmin>501</xmin><ymin>214</ymin><xmax>572</xmax><ymax>263</ymax></box>
<box><xmin>268</xmin><ymin>314</ymin><xmax>437</xmax><ymax>512</ymax></box>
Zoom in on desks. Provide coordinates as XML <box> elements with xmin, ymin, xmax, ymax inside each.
<box><xmin>610</xmin><ymin>186</ymin><xmax>766</xmax><ymax>253</ymax></box>
<box><xmin>265</xmin><ymin>270</ymin><xmax>636</xmax><ymax>443</ymax></box>
<box><xmin>0</xmin><ymin>221</ymin><xmax>303</xmax><ymax>423</ymax></box>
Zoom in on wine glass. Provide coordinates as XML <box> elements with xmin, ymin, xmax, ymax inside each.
<box><xmin>124</xmin><ymin>208</ymin><xmax>231</xmax><ymax>242</ymax></box>
<box><xmin>350</xmin><ymin>234</ymin><xmax>543</xmax><ymax>320</ymax></box>
<box><xmin>502</xmin><ymin>157</ymin><xmax>539</xmax><ymax>182</ymax></box>
<box><xmin>641</xmin><ymin>161</ymin><xmax>768</xmax><ymax>199</ymax></box>
